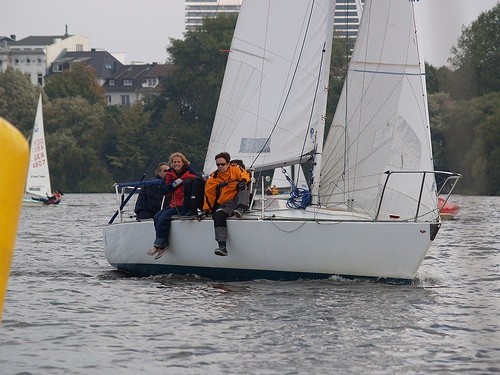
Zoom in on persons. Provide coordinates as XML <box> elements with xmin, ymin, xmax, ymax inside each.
<box><xmin>230</xmin><ymin>159</ymin><xmax>255</xmax><ymax>207</ymax></box>
<box><xmin>134</xmin><ymin>161</ymin><xmax>170</xmax><ymax>220</ymax></box>
<box><xmin>197</xmin><ymin>152</ymin><xmax>251</xmax><ymax>255</ymax></box>
<box><xmin>146</xmin><ymin>152</ymin><xmax>205</xmax><ymax>260</ymax></box>
<box><xmin>31</xmin><ymin>188</ymin><xmax>64</xmax><ymax>204</ymax></box>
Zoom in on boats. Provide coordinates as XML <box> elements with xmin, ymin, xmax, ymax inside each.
<box><xmin>437</xmin><ymin>198</ymin><xmax>461</xmax><ymax>220</ymax></box>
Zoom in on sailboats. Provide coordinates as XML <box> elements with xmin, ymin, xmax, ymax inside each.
<box><xmin>254</xmin><ymin>163</ymin><xmax>309</xmax><ymax>210</ymax></box>
<box><xmin>24</xmin><ymin>92</ymin><xmax>63</xmax><ymax>206</ymax></box>
<box><xmin>102</xmin><ymin>0</ymin><xmax>463</xmax><ymax>282</ymax></box>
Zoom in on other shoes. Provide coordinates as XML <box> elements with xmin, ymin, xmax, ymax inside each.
<box><xmin>179</xmin><ymin>209</ymin><xmax>198</xmax><ymax>217</ymax></box>
<box><xmin>233</xmin><ymin>203</ymin><xmax>249</xmax><ymax>218</ymax></box>
<box><xmin>214</xmin><ymin>242</ymin><xmax>228</xmax><ymax>256</ymax></box>
<box><xmin>154</xmin><ymin>247</ymin><xmax>169</xmax><ymax>259</ymax></box>
<box><xmin>146</xmin><ymin>247</ymin><xmax>160</xmax><ymax>256</ymax></box>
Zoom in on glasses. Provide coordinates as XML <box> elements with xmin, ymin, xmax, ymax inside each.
<box><xmin>215</xmin><ymin>162</ymin><xmax>227</xmax><ymax>166</ymax></box>
<box><xmin>163</xmin><ymin>169</ymin><xmax>170</xmax><ymax>172</ymax></box>
